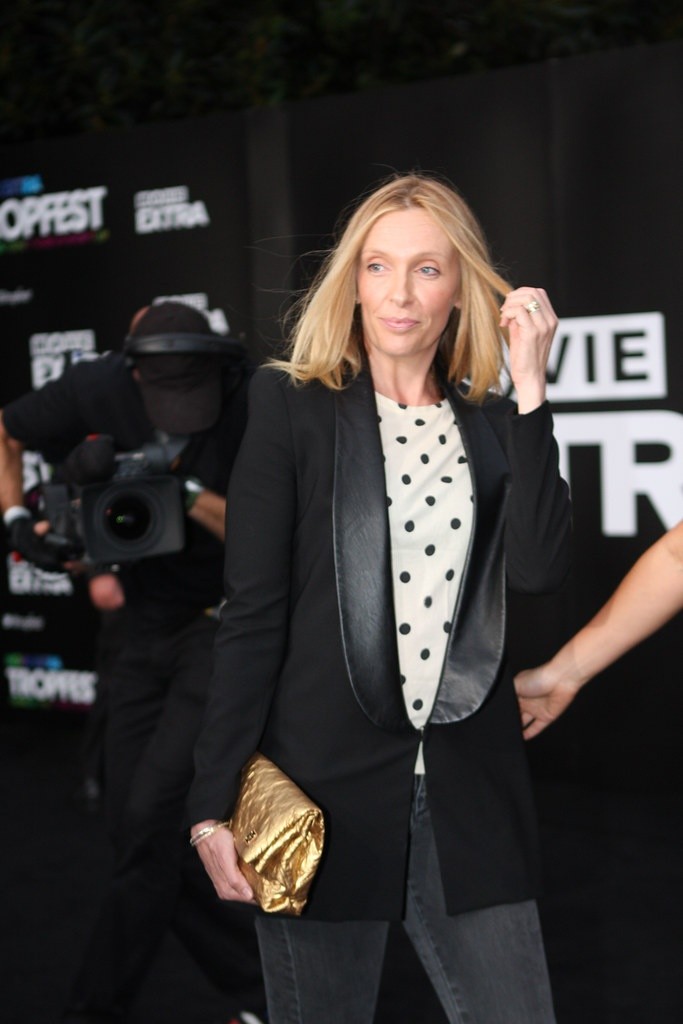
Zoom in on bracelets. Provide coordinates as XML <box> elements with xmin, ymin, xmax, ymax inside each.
<box><xmin>174</xmin><ymin>468</ymin><xmax>202</xmax><ymax>511</ymax></box>
<box><xmin>4</xmin><ymin>506</ymin><xmax>30</xmax><ymax>525</ymax></box>
<box><xmin>189</xmin><ymin>819</ymin><xmax>229</xmax><ymax>847</ymax></box>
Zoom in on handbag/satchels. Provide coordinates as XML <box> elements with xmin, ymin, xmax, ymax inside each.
<box><xmin>227</xmin><ymin>752</ymin><xmax>324</xmax><ymax>916</ymax></box>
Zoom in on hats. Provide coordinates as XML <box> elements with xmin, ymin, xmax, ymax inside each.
<box><xmin>124</xmin><ymin>302</ymin><xmax>224</xmax><ymax>435</ymax></box>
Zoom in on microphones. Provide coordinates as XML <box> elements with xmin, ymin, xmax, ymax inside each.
<box><xmin>65</xmin><ymin>436</ymin><xmax>114</xmax><ymax>482</ymax></box>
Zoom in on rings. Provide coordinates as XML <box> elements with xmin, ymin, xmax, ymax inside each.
<box><xmin>526</xmin><ymin>301</ymin><xmax>540</xmax><ymax>313</ymax></box>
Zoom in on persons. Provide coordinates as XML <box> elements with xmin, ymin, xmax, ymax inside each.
<box><xmin>180</xmin><ymin>171</ymin><xmax>582</xmax><ymax>1024</ymax></box>
<box><xmin>513</xmin><ymin>520</ymin><xmax>683</xmax><ymax>740</ymax></box>
<box><xmin>0</xmin><ymin>302</ymin><xmax>264</xmax><ymax>1024</ymax></box>
